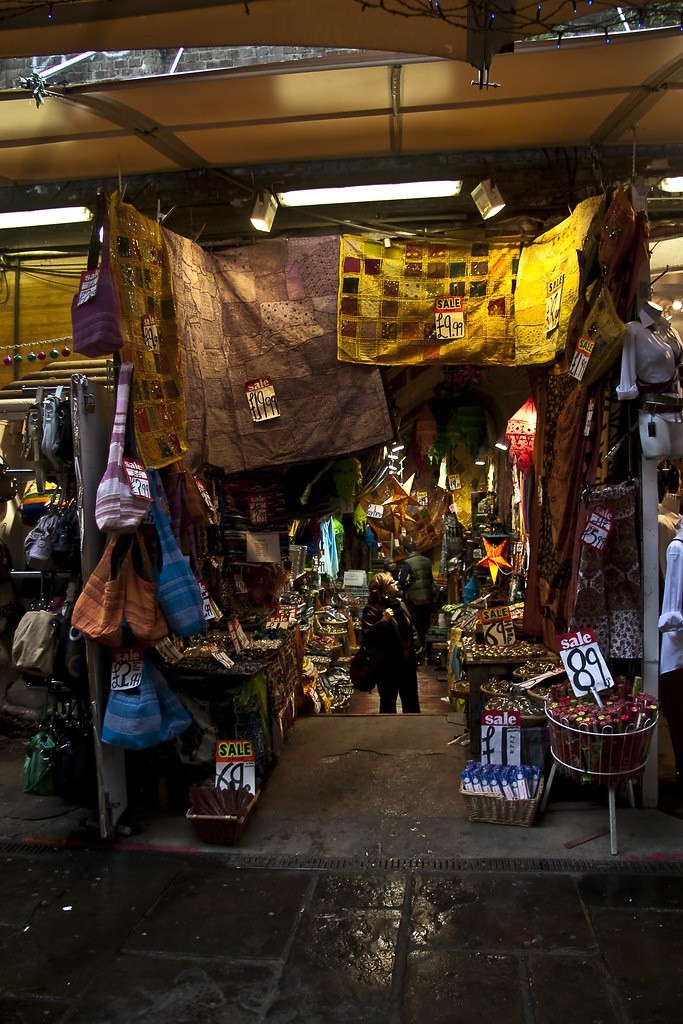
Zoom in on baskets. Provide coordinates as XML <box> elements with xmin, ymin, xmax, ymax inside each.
<box><xmin>459</xmin><ymin>773</ymin><xmax>545</xmax><ymax>828</ymax></box>
<box><xmin>186</xmin><ymin>786</ymin><xmax>262</xmax><ymax>846</ymax></box>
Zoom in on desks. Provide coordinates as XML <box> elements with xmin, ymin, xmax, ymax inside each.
<box><xmin>467</xmin><ymin>644</ymin><xmax>561</xmax><ymax>755</ymax></box>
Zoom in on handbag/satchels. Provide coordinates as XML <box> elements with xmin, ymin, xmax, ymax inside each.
<box><xmin>22</xmin><ymin>683</ymin><xmax>219</xmax><ymax>822</ymax></box>
<box><xmin>348</xmin><ymin>596</ymin><xmax>426</xmax><ymax>696</ymax></box>
<box><xmin>99</xmin><ymin>618</ymin><xmax>198</xmax><ymax>751</ymax></box>
<box><xmin>0</xmin><ymin>673</ymin><xmax>51</xmax><ymax>740</ymax></box>
<box><xmin>0</xmin><ymin>567</ymin><xmax>88</xmax><ymax>693</ymax></box>
<box><xmin>18</xmin><ymin>384</ymin><xmax>74</xmax><ymax>468</ymax></box>
<box><xmin>69</xmin><ymin>467</ymin><xmax>208</xmax><ymax>651</ymax></box>
<box><xmin>71</xmin><ymin>185</ymin><xmax>125</xmax><ymax>359</ymax></box>
<box><xmin>94</xmin><ymin>362</ymin><xmax>152</xmax><ymax>532</ymax></box>
<box><xmin>0</xmin><ymin>466</ymin><xmax>81</xmax><ymax>584</ymax></box>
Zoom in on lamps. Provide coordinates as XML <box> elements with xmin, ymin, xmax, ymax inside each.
<box><xmin>471</xmin><ymin>178</ymin><xmax>506</xmax><ymax>220</ymax></box>
<box><xmin>390</xmin><ymin>428</ymin><xmax>404</xmax><ymax>453</ymax></box>
<box><xmin>495</xmin><ymin>429</ymin><xmax>511</xmax><ymax>450</ymax></box>
<box><xmin>250</xmin><ymin>189</ymin><xmax>278</xmax><ymax>232</ymax></box>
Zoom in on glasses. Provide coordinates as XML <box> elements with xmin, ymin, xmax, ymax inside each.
<box><xmin>385</xmin><ymin>578</ymin><xmax>396</xmax><ymax>588</ymax></box>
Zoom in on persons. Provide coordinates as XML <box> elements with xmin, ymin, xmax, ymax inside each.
<box><xmin>654</xmin><ymin>500</ymin><xmax>682</xmax><ymax>822</ymax></box>
<box><xmin>615</xmin><ymin>301</ymin><xmax>683</xmax><ymax>460</ymax></box>
<box><xmin>360</xmin><ymin>539</ymin><xmax>435</xmax><ymax>713</ymax></box>
<box><xmin>657</xmin><ymin>492</ymin><xmax>683</xmax><ymax>575</ymax></box>
